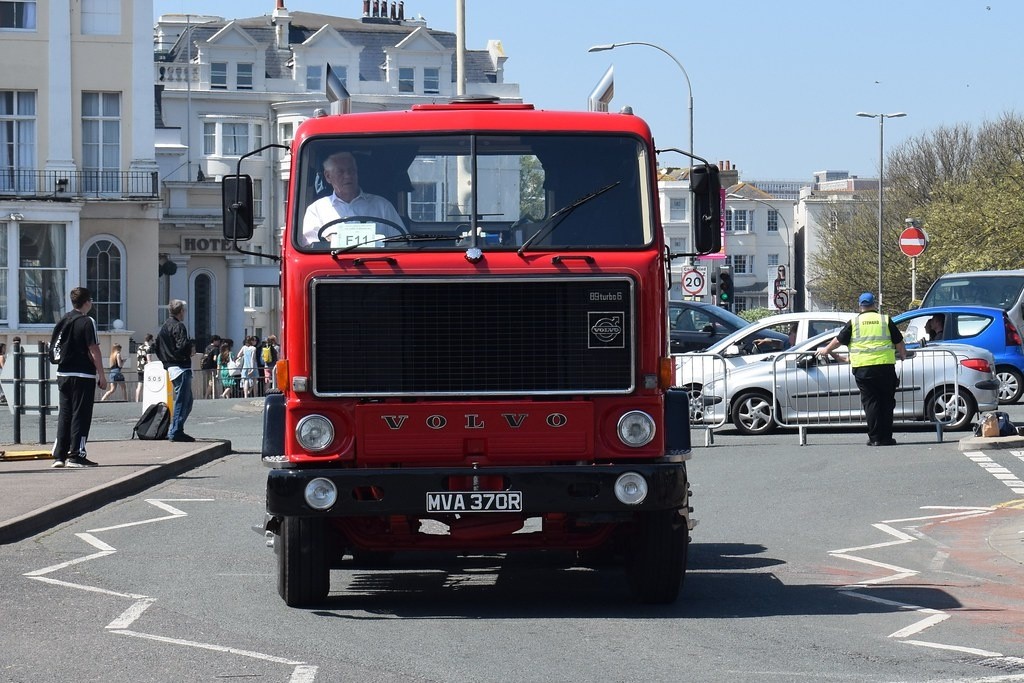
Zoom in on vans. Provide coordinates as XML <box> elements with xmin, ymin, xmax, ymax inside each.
<box><xmin>905</xmin><ymin>269</ymin><xmax>1024</xmax><ymax>349</ymax></box>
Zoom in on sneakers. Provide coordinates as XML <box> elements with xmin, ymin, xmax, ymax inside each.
<box><xmin>67</xmin><ymin>456</ymin><xmax>98</xmax><ymax>468</ymax></box>
<box><xmin>51</xmin><ymin>459</ymin><xmax>66</xmax><ymax>468</ymax></box>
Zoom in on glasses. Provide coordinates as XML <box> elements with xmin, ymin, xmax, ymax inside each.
<box><xmin>88</xmin><ymin>297</ymin><xmax>93</xmax><ymax>302</ymax></box>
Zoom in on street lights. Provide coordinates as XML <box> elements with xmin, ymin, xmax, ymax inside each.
<box><xmin>856</xmin><ymin>111</ymin><xmax>908</xmax><ymax>315</ymax></box>
<box><xmin>588</xmin><ymin>40</ymin><xmax>697</xmax><ymax>301</ymax></box>
<box><xmin>749</xmin><ymin>197</ymin><xmax>791</xmax><ymax>313</ymax></box>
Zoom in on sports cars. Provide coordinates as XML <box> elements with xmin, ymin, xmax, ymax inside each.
<box><xmin>698</xmin><ymin>323</ymin><xmax>1002</xmax><ymax>435</ymax></box>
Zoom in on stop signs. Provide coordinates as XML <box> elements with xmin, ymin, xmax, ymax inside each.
<box><xmin>899</xmin><ymin>227</ymin><xmax>926</xmax><ymax>258</ymax></box>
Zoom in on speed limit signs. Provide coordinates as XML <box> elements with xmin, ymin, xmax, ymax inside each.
<box><xmin>682</xmin><ymin>270</ymin><xmax>705</xmax><ymax>294</ymax></box>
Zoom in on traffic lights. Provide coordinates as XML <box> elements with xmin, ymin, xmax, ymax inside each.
<box><xmin>720</xmin><ymin>266</ymin><xmax>734</xmax><ymax>305</ymax></box>
<box><xmin>711</xmin><ymin>267</ymin><xmax>721</xmax><ymax>307</ymax></box>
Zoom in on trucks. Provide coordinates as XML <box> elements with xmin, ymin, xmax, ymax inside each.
<box><xmin>221</xmin><ymin>96</ymin><xmax>720</xmax><ymax>615</ymax></box>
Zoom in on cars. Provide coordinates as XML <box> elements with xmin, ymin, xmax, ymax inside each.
<box><xmin>883</xmin><ymin>305</ymin><xmax>1024</xmax><ymax>406</ymax></box>
<box><xmin>667</xmin><ymin>299</ymin><xmax>792</xmax><ymax>355</ymax></box>
<box><xmin>673</xmin><ymin>311</ymin><xmax>906</xmax><ymax>427</ymax></box>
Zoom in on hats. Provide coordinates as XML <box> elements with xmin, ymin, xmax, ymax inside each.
<box><xmin>859</xmin><ymin>292</ymin><xmax>878</xmax><ymax>306</ymax></box>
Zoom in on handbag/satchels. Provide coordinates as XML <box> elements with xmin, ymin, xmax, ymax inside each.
<box><xmin>227</xmin><ymin>352</ymin><xmax>240</xmax><ymax>376</ymax></box>
<box><xmin>235</xmin><ymin>356</ymin><xmax>243</xmax><ymax>369</ymax></box>
<box><xmin>114</xmin><ymin>373</ymin><xmax>125</xmax><ymax>381</ymax></box>
<box><xmin>48</xmin><ymin>312</ymin><xmax>89</xmax><ymax>365</ymax></box>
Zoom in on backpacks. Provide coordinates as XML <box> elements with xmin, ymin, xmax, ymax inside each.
<box><xmin>130</xmin><ymin>402</ymin><xmax>171</xmax><ymax>440</ymax></box>
<box><xmin>261</xmin><ymin>344</ymin><xmax>272</xmax><ymax>363</ymax></box>
<box><xmin>200</xmin><ymin>347</ymin><xmax>216</xmax><ymax>370</ymax></box>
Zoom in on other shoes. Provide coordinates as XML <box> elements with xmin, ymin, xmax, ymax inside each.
<box><xmin>866</xmin><ymin>440</ymin><xmax>896</xmax><ymax>446</ymax></box>
<box><xmin>170</xmin><ymin>434</ymin><xmax>196</xmax><ymax>442</ymax></box>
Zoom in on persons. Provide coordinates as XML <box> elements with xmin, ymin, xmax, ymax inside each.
<box><xmin>135</xmin><ymin>334</ymin><xmax>153</xmax><ymax>402</ymax></box>
<box><xmin>302</xmin><ymin>152</ymin><xmax>408</xmax><ymax>248</ymax></box>
<box><xmin>204</xmin><ymin>334</ymin><xmax>280</xmax><ymax>399</ymax></box>
<box><xmin>925</xmin><ymin>314</ymin><xmax>945</xmax><ymax>341</ymax></box>
<box><xmin>0</xmin><ymin>343</ymin><xmax>7</xmax><ymax>369</ymax></box>
<box><xmin>815</xmin><ymin>293</ymin><xmax>907</xmax><ymax>446</ymax></box>
<box><xmin>754</xmin><ymin>324</ymin><xmax>797</xmax><ymax>351</ymax></box>
<box><xmin>829</xmin><ymin>351</ymin><xmax>848</xmax><ymax>362</ymax></box>
<box><xmin>102</xmin><ymin>344</ymin><xmax>127</xmax><ymax>400</ymax></box>
<box><xmin>155</xmin><ymin>299</ymin><xmax>195</xmax><ymax>442</ymax></box>
<box><xmin>51</xmin><ymin>286</ymin><xmax>107</xmax><ymax>467</ymax></box>
